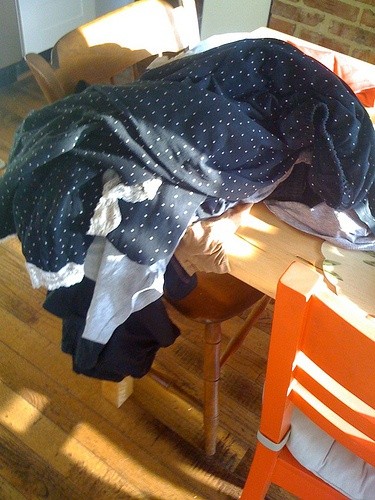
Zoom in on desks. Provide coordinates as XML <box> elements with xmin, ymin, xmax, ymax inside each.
<box><xmin>223</xmin><ymin>24</ymin><xmax>375</xmax><ymax>318</ymax></box>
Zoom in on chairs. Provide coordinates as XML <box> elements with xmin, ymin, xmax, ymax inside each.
<box><xmin>239</xmin><ymin>260</ymin><xmax>375</xmax><ymax>500</ymax></box>
<box><xmin>21</xmin><ymin>0</ymin><xmax>271</xmax><ymax>463</ymax></box>
